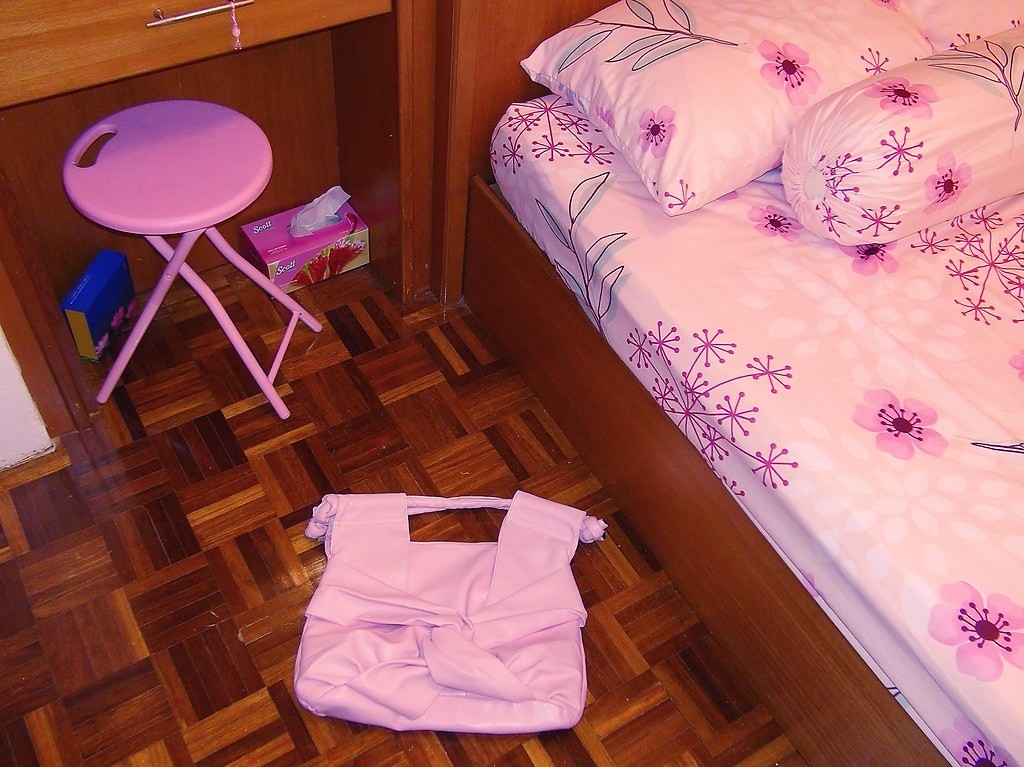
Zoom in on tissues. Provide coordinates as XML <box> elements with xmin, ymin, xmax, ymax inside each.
<box><xmin>240</xmin><ymin>187</ymin><xmax>371</xmax><ymax>299</ymax></box>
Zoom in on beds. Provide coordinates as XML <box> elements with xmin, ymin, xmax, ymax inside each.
<box><xmin>464</xmin><ymin>91</ymin><xmax>1023</xmax><ymax>766</ymax></box>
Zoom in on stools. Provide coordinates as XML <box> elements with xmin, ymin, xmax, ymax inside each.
<box><xmin>61</xmin><ymin>99</ymin><xmax>323</xmax><ymax>419</ymax></box>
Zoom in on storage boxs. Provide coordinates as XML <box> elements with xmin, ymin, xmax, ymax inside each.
<box><xmin>63</xmin><ymin>247</ymin><xmax>140</xmax><ymax>364</ymax></box>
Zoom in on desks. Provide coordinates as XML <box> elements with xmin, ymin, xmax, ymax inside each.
<box><xmin>0</xmin><ymin>0</ymin><xmax>416</xmax><ymax>306</ymax></box>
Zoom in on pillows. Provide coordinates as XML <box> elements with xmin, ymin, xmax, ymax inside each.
<box><xmin>781</xmin><ymin>22</ymin><xmax>1024</xmax><ymax>247</ymax></box>
<box><xmin>515</xmin><ymin>0</ymin><xmax>1024</xmax><ymax>217</ymax></box>
